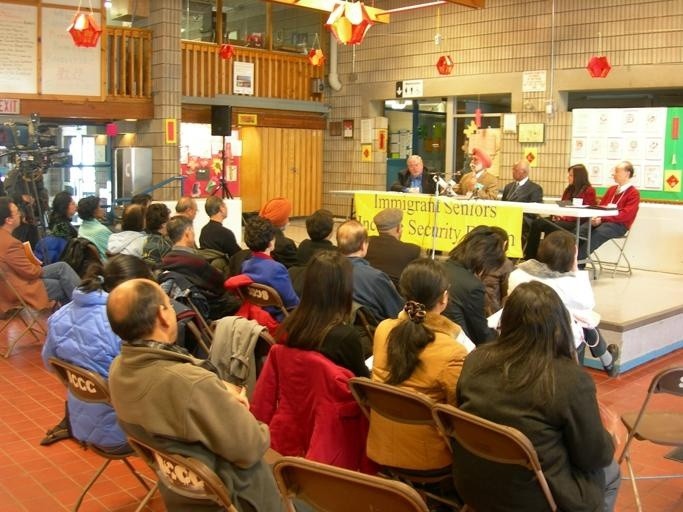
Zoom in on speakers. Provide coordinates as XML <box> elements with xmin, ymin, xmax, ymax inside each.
<box><xmin>211</xmin><ymin>106</ymin><xmax>232</xmax><ymax>136</ymax></box>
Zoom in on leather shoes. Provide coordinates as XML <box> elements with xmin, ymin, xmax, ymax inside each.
<box><xmin>604</xmin><ymin>343</ymin><xmax>618</xmax><ymax>375</ymax></box>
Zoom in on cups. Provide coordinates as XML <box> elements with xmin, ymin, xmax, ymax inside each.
<box><xmin>573</xmin><ymin>198</ymin><xmax>584</xmax><ymax>206</ymax></box>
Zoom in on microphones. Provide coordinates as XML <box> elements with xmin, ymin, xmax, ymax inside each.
<box><xmin>458</xmin><ymin>167</ymin><xmax>468</xmax><ymax>176</ymax></box>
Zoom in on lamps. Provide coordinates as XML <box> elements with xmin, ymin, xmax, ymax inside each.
<box><xmin>69</xmin><ymin>0</ymin><xmax>103</xmax><ymax>46</ymax></box>
<box><xmin>219</xmin><ymin>0</ymin><xmax>237</xmax><ymax>60</ymax></box>
<box><xmin>325</xmin><ymin>0</ymin><xmax>375</xmax><ymax>46</ymax></box>
<box><xmin>437</xmin><ymin>0</ymin><xmax>453</xmax><ymax>74</ymax></box>
<box><xmin>587</xmin><ymin>0</ymin><xmax>611</xmax><ymax>77</ymax></box>
<box><xmin>308</xmin><ymin>0</ymin><xmax>325</xmax><ymax>67</ymax></box>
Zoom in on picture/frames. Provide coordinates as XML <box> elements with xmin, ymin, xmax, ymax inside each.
<box><xmin>518</xmin><ymin>122</ymin><xmax>545</xmax><ymax>143</ymax></box>
<box><xmin>343</xmin><ymin>120</ymin><xmax>353</xmax><ymax>137</ymax></box>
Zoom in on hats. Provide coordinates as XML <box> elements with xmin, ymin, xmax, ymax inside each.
<box><xmin>259</xmin><ymin>199</ymin><xmax>292</xmax><ymax>227</ymax></box>
<box><xmin>471</xmin><ymin>146</ymin><xmax>492</xmax><ymax>167</ymax></box>
<box><xmin>373</xmin><ymin>208</ymin><xmax>403</xmax><ymax>230</ymax></box>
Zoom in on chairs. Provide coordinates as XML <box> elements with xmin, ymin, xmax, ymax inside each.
<box><xmin>595</xmin><ymin>229</ymin><xmax>633</xmax><ymax>278</ymax></box>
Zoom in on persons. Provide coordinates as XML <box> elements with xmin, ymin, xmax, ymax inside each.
<box><xmin>501</xmin><ymin>161</ymin><xmax>543</xmax><ymax>265</ymax></box>
<box><xmin>3</xmin><ymin>166</ymin><xmax>49</xmax><ymax>251</ymax></box>
<box><xmin>391</xmin><ymin>154</ymin><xmax>440</xmax><ymax>193</ymax></box>
<box><xmin>106</xmin><ymin>277</ymin><xmax>315</xmax><ymax>512</ymax></box>
<box><xmin>448</xmin><ymin>281</ymin><xmax>621</xmax><ymax>511</ymax></box>
<box><xmin>0</xmin><ymin>196</ymin><xmax>83</xmax><ymax>313</ymax></box>
<box><xmin>526</xmin><ymin>164</ymin><xmax>598</xmax><ymax>260</ymax></box>
<box><xmin>41</xmin><ymin>191</ymin><xmax>619</xmax><ymax>477</ymax></box>
<box><xmin>452</xmin><ymin>148</ymin><xmax>499</xmax><ymax>200</ymax></box>
<box><xmin>571</xmin><ymin>160</ymin><xmax>640</xmax><ymax>271</ymax></box>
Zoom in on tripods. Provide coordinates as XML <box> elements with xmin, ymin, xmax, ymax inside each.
<box><xmin>201</xmin><ymin>179</ymin><xmax>250</xmax><ymax>232</ymax></box>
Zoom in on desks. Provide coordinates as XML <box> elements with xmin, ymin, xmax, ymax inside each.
<box><xmin>331</xmin><ymin>190</ymin><xmax>619</xmax><ymax>279</ymax></box>
